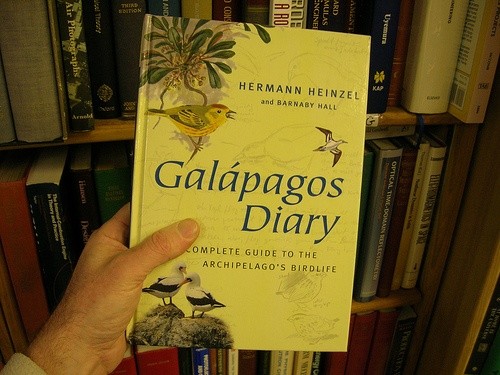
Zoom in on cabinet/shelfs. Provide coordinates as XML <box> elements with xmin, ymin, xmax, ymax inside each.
<box><xmin>0</xmin><ymin>0</ymin><xmax>500</xmax><ymax>375</ymax></box>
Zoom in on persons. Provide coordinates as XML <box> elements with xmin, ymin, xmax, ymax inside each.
<box><xmin>0</xmin><ymin>203</ymin><xmax>199</xmax><ymax>375</ymax></box>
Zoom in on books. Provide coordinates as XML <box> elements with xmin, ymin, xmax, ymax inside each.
<box><xmin>0</xmin><ymin>127</ymin><xmax>500</xmax><ymax>375</ymax></box>
<box><xmin>125</xmin><ymin>14</ymin><xmax>371</xmax><ymax>352</ymax></box>
<box><xmin>0</xmin><ymin>0</ymin><xmax>500</xmax><ymax>143</ymax></box>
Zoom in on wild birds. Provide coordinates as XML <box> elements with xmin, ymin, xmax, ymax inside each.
<box><xmin>142</xmin><ymin>261</ymin><xmax>187</xmax><ymax>307</ymax></box>
<box><xmin>177</xmin><ymin>271</ymin><xmax>227</xmax><ymax>319</ymax></box>
